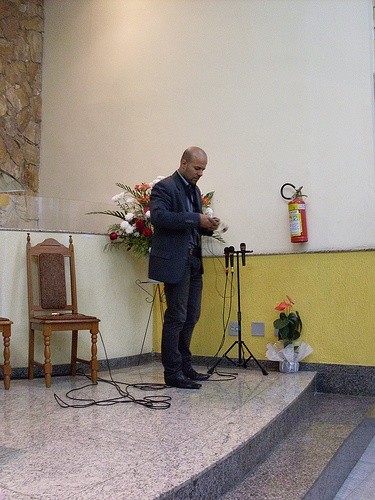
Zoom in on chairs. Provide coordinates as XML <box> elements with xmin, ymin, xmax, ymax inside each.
<box><xmin>27</xmin><ymin>233</ymin><xmax>101</xmax><ymax>388</ymax></box>
<box><xmin>0</xmin><ymin>317</ymin><xmax>14</xmax><ymax>390</ymax></box>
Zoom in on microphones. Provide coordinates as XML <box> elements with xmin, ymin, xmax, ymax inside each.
<box><xmin>224</xmin><ymin>247</ymin><xmax>229</xmax><ymax>276</ymax></box>
<box><xmin>229</xmin><ymin>246</ymin><xmax>234</xmax><ymax>274</ymax></box>
<box><xmin>240</xmin><ymin>243</ymin><xmax>246</xmax><ymax>266</ymax></box>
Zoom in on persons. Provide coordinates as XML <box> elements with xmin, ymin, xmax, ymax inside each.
<box><xmin>146</xmin><ymin>145</ymin><xmax>221</xmax><ymax>390</ymax></box>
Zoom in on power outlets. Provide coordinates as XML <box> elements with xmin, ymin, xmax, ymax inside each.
<box><xmin>228</xmin><ymin>322</ymin><xmax>239</xmax><ymax>336</ymax></box>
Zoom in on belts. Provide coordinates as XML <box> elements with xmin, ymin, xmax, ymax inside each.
<box><xmin>188</xmin><ymin>248</ymin><xmax>200</xmax><ymax>255</ymax></box>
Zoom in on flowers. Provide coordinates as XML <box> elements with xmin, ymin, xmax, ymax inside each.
<box><xmin>86</xmin><ymin>176</ymin><xmax>228</xmax><ymax>256</ymax></box>
<box><xmin>274</xmin><ymin>295</ymin><xmax>302</xmax><ymax>340</ymax></box>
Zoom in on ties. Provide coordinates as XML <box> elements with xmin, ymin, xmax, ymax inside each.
<box><xmin>176</xmin><ymin>170</ymin><xmax>201</xmax><ymax>214</ymax></box>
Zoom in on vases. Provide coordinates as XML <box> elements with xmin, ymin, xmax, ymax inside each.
<box><xmin>266</xmin><ymin>340</ymin><xmax>313</xmax><ymax>373</ymax></box>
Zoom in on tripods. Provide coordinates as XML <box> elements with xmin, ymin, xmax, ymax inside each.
<box><xmin>206</xmin><ymin>251</ymin><xmax>268</xmax><ymax>375</ymax></box>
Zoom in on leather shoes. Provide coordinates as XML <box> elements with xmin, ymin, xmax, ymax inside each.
<box><xmin>165</xmin><ymin>376</ymin><xmax>202</xmax><ymax>389</ymax></box>
<box><xmin>184</xmin><ymin>368</ymin><xmax>210</xmax><ymax>381</ymax></box>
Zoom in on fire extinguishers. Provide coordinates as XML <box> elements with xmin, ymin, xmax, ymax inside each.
<box><xmin>280</xmin><ymin>183</ymin><xmax>308</xmax><ymax>243</ymax></box>
